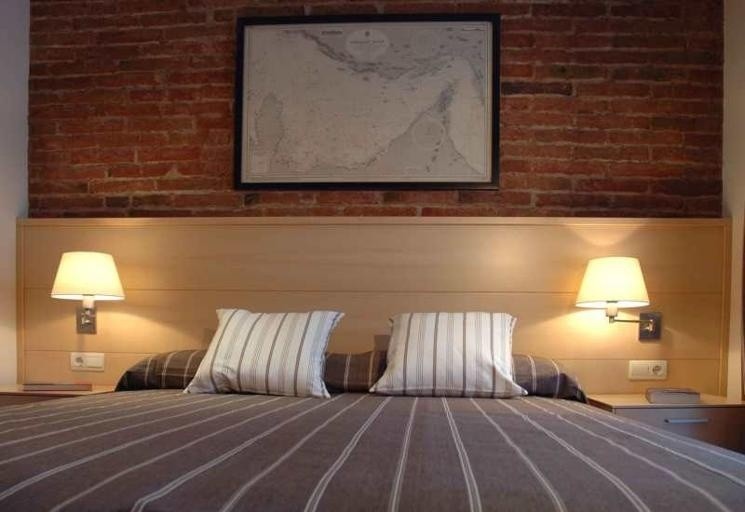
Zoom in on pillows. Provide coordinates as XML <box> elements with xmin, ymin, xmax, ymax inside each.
<box><xmin>112</xmin><ymin>347</ymin><xmax>583</xmax><ymax>400</ymax></box>
<box><xmin>369</xmin><ymin>309</ymin><xmax>530</xmax><ymax>398</ymax></box>
<box><xmin>180</xmin><ymin>307</ymin><xmax>348</xmax><ymax>400</ymax></box>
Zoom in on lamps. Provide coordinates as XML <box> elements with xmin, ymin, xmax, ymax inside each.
<box><xmin>48</xmin><ymin>251</ymin><xmax>125</xmax><ymax>334</ymax></box>
<box><xmin>573</xmin><ymin>255</ymin><xmax>662</xmax><ymax>341</ymax></box>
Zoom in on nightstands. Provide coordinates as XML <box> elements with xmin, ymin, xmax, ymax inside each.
<box><xmin>585</xmin><ymin>391</ymin><xmax>745</xmax><ymax>454</ymax></box>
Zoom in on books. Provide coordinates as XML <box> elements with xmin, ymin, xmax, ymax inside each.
<box><xmin>645</xmin><ymin>388</ymin><xmax>701</xmax><ymax>405</ymax></box>
<box><xmin>21</xmin><ymin>377</ymin><xmax>92</xmax><ymax>392</ymax></box>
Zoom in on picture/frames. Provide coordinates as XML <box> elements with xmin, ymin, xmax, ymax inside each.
<box><xmin>232</xmin><ymin>13</ymin><xmax>499</xmax><ymax>192</ymax></box>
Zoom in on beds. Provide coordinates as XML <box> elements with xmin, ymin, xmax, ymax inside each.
<box><xmin>0</xmin><ymin>388</ymin><xmax>745</xmax><ymax>512</ymax></box>
